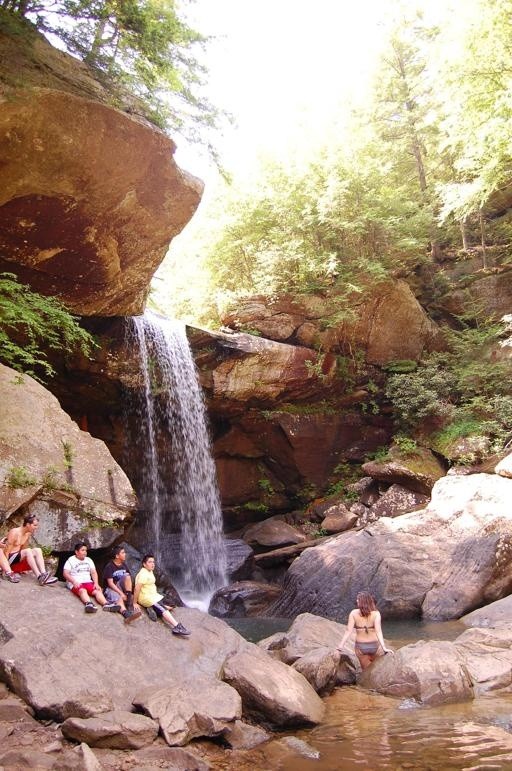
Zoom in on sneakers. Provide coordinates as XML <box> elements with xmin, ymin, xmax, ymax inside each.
<box><xmin>173</xmin><ymin>623</ymin><xmax>190</xmax><ymax>635</ymax></box>
<box><xmin>147</xmin><ymin>607</ymin><xmax>157</xmax><ymax>621</ymax></box>
<box><xmin>103</xmin><ymin>604</ymin><xmax>121</xmax><ymax>612</ymax></box>
<box><xmin>37</xmin><ymin>571</ymin><xmax>58</xmax><ymax>585</ymax></box>
<box><xmin>84</xmin><ymin>601</ymin><xmax>97</xmax><ymax>613</ymax></box>
<box><xmin>5</xmin><ymin>571</ymin><xmax>20</xmax><ymax>583</ymax></box>
<box><xmin>125</xmin><ymin>611</ymin><xmax>142</xmax><ymax>623</ymax></box>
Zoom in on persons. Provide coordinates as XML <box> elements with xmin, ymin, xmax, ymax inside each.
<box><xmin>0</xmin><ymin>514</ymin><xmax>59</xmax><ymax>588</ymax></box>
<box><xmin>335</xmin><ymin>591</ymin><xmax>395</xmax><ymax>671</ymax></box>
<box><xmin>102</xmin><ymin>545</ymin><xmax>142</xmax><ymax>624</ymax></box>
<box><xmin>63</xmin><ymin>542</ymin><xmax>122</xmax><ymax>614</ymax></box>
<box><xmin>132</xmin><ymin>554</ymin><xmax>192</xmax><ymax>636</ymax></box>
<box><xmin>0</xmin><ymin>537</ymin><xmax>21</xmax><ymax>584</ymax></box>
